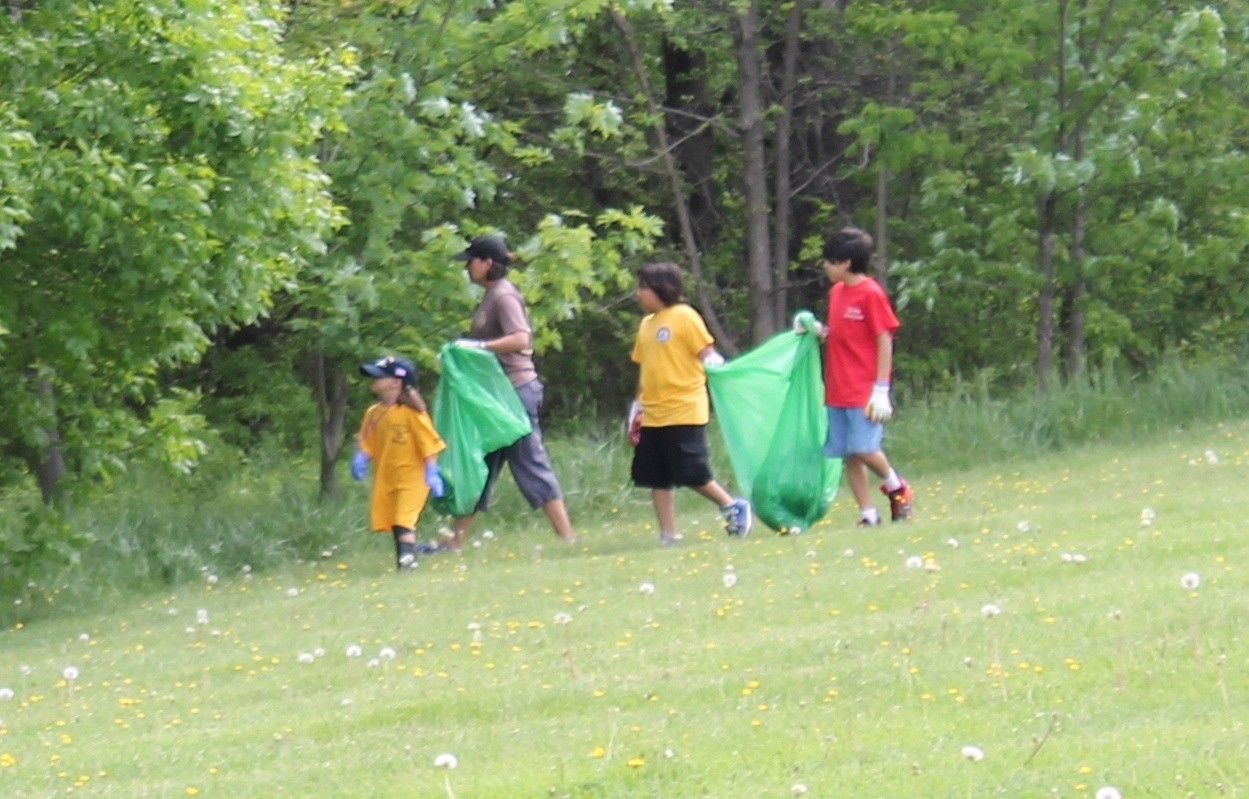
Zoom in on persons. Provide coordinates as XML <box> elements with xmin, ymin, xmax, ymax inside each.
<box><xmin>350</xmin><ymin>354</ymin><xmax>446</xmax><ymax>569</ymax></box>
<box><xmin>628</xmin><ymin>261</ymin><xmax>752</xmax><ymax>544</ymax></box>
<box><xmin>417</xmin><ymin>236</ymin><xmax>572</xmax><ymax>554</ymax></box>
<box><xmin>795</xmin><ymin>228</ymin><xmax>917</xmax><ymax>527</ymax></box>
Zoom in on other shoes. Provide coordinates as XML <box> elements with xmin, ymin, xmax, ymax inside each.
<box><xmin>420</xmin><ymin>545</ymin><xmax>460</xmax><ymax>557</ymax></box>
<box><xmin>720</xmin><ymin>500</ymin><xmax>753</xmax><ymax>540</ymax></box>
<box><xmin>398</xmin><ymin>551</ymin><xmax>420</xmax><ymax>571</ymax></box>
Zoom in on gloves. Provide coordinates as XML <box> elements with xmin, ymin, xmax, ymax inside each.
<box><xmin>454</xmin><ymin>339</ymin><xmax>480</xmax><ymax>350</ymax></box>
<box><xmin>794</xmin><ymin>311</ymin><xmax>821</xmax><ymax>337</ymax></box>
<box><xmin>426</xmin><ymin>466</ymin><xmax>444</xmax><ymax>499</ymax></box>
<box><xmin>352</xmin><ymin>454</ymin><xmax>366</xmax><ymax>479</ymax></box>
<box><xmin>628</xmin><ymin>402</ymin><xmax>643</xmax><ymax>446</ymax></box>
<box><xmin>864</xmin><ymin>385</ymin><xmax>893</xmax><ymax>423</ymax></box>
<box><xmin>705</xmin><ymin>353</ymin><xmax>723</xmax><ymax>369</ymax></box>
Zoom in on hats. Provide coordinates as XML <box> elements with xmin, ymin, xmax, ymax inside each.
<box><xmin>358</xmin><ymin>355</ymin><xmax>417</xmax><ymax>384</ymax></box>
<box><xmin>448</xmin><ymin>234</ymin><xmax>512</xmax><ymax>265</ymax></box>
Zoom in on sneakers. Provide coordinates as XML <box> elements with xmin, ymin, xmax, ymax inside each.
<box><xmin>857</xmin><ymin>516</ymin><xmax>881</xmax><ymax>530</ymax></box>
<box><xmin>880</xmin><ymin>478</ymin><xmax>914</xmax><ymax>522</ymax></box>
